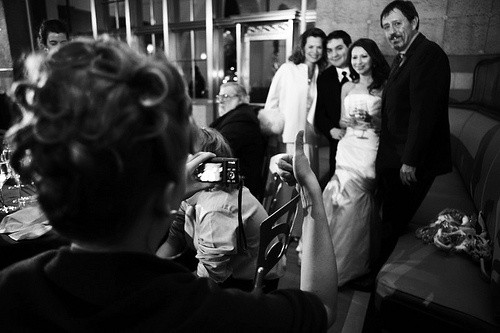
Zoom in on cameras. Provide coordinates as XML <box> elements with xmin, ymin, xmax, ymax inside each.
<box><xmin>194</xmin><ymin>157</ymin><xmax>239</xmax><ymax>186</ymax></box>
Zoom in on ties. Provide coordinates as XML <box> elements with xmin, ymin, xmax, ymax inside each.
<box><xmin>385</xmin><ymin>51</ymin><xmax>403</xmax><ymax>85</ymax></box>
<box><xmin>341</xmin><ymin>70</ymin><xmax>349</xmax><ymax>95</ymax></box>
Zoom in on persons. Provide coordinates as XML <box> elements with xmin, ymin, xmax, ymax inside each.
<box><xmin>222</xmin><ymin>30</ymin><xmax>238</xmax><ymax>82</ymax></box>
<box><xmin>321</xmin><ymin>36</ymin><xmax>393</xmax><ymax>290</ymax></box>
<box><xmin>258</xmin><ymin>27</ymin><xmax>329</xmax><ymax>243</ymax></box>
<box><xmin>187</xmin><ymin>65</ymin><xmax>208</xmax><ymax>99</ymax></box>
<box><xmin>155</xmin><ymin>124</ymin><xmax>289</xmax><ymax>293</ymax></box>
<box><xmin>130</xmin><ymin>26</ymin><xmax>149</xmax><ymax>57</ymax></box>
<box><xmin>0</xmin><ymin>32</ymin><xmax>340</xmax><ymax>333</ymax></box>
<box><xmin>206</xmin><ymin>82</ymin><xmax>272</xmax><ymax>204</ymax></box>
<box><xmin>372</xmin><ymin>1</ymin><xmax>454</xmax><ymax>260</ymax></box>
<box><xmin>36</xmin><ymin>18</ymin><xmax>72</xmax><ymax>55</ymax></box>
<box><xmin>313</xmin><ymin>29</ymin><xmax>356</xmax><ymax>191</ymax></box>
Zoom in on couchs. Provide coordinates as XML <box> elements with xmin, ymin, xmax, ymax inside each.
<box><xmin>378</xmin><ymin>107</ymin><xmax>500</xmax><ymax>332</ymax></box>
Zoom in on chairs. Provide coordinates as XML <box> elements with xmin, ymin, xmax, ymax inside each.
<box><xmin>247</xmin><ymin>193</ymin><xmax>302</xmax><ymax>292</ymax></box>
